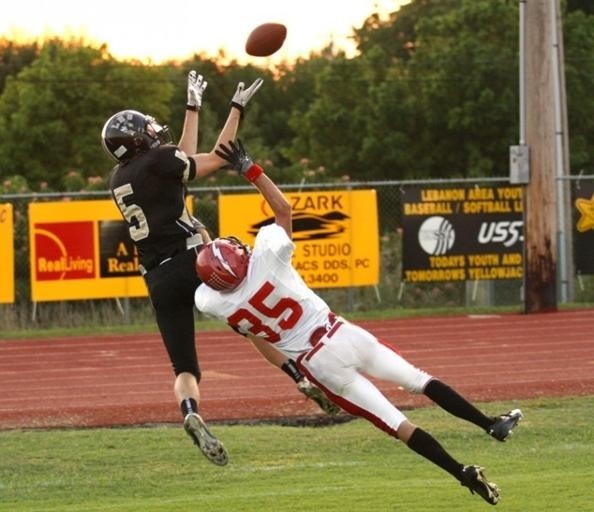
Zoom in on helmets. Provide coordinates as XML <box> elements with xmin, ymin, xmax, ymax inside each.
<box><xmin>192</xmin><ymin>235</ymin><xmax>251</xmax><ymax>294</ymax></box>
<box><xmin>99</xmin><ymin>108</ymin><xmax>174</xmax><ymax>165</ymax></box>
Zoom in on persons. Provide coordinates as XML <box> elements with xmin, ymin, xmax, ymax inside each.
<box><xmin>176</xmin><ymin>139</ymin><xmax>521</xmax><ymax>506</ymax></box>
<box><xmin>98</xmin><ymin>70</ymin><xmax>268</xmax><ymax>467</ymax></box>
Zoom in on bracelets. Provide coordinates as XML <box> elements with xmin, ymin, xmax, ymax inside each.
<box><xmin>243</xmin><ymin>166</ymin><xmax>261</xmax><ymax>185</ymax></box>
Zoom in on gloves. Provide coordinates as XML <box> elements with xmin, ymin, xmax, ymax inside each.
<box><xmin>184</xmin><ymin>70</ymin><xmax>209</xmax><ymax>112</ymax></box>
<box><xmin>217</xmin><ymin>137</ymin><xmax>257</xmax><ymax>178</ymax></box>
<box><xmin>227</xmin><ymin>77</ymin><xmax>266</xmax><ymax>112</ymax></box>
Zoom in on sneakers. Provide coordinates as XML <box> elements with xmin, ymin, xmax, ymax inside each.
<box><xmin>486</xmin><ymin>407</ymin><xmax>524</xmax><ymax>444</ymax></box>
<box><xmin>184</xmin><ymin>412</ymin><xmax>229</xmax><ymax>466</ymax></box>
<box><xmin>461</xmin><ymin>463</ymin><xmax>501</xmax><ymax>506</ymax></box>
<box><xmin>294</xmin><ymin>379</ymin><xmax>340</xmax><ymax>417</ymax></box>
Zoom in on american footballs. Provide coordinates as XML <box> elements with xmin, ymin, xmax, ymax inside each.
<box><xmin>245</xmin><ymin>22</ymin><xmax>287</xmax><ymax>57</ymax></box>
